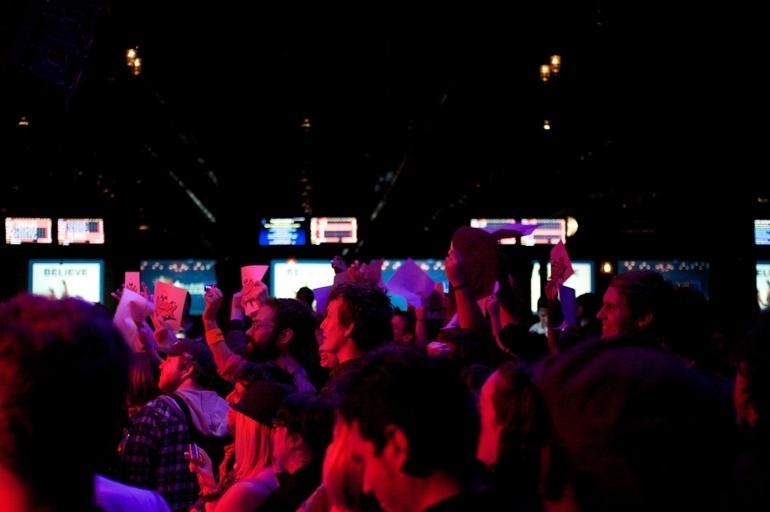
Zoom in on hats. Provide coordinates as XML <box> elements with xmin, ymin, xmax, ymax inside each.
<box><xmin>157</xmin><ymin>337</ymin><xmax>215</xmax><ymax>372</ymax></box>
<box><xmin>229</xmin><ymin>379</ymin><xmax>288</xmax><ymax>428</ymax></box>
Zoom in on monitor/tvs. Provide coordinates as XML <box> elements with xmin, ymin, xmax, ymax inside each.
<box><xmin>269</xmin><ymin>259</ymin><xmax>336</xmax><ymax>312</ymax></box>
<box><xmin>530</xmin><ymin>260</ymin><xmax>596</xmax><ymax>315</ymax></box>
<box><xmin>258</xmin><ymin>217</ymin><xmax>307</xmax><ymax>247</ymax></box>
<box><xmin>310</xmin><ymin>216</ymin><xmax>358</xmax><ymax>246</ymax></box>
<box><xmin>28</xmin><ymin>258</ymin><xmax>105</xmax><ymax>305</ymax></box>
<box><xmin>140</xmin><ymin>258</ymin><xmax>218</xmax><ymax>317</ymax></box>
<box><xmin>519</xmin><ymin>219</ymin><xmax>566</xmax><ymax>247</ymax></box>
<box><xmin>56</xmin><ymin>216</ymin><xmax>106</xmax><ymax>247</ymax></box>
<box><xmin>470</xmin><ymin>219</ymin><xmax>517</xmax><ymax>246</ymax></box>
<box><xmin>371</xmin><ymin>259</ymin><xmax>450</xmax><ymax>311</ymax></box>
<box><xmin>4</xmin><ymin>217</ymin><xmax>53</xmax><ymax>246</ymax></box>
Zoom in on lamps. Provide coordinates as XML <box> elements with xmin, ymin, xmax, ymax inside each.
<box><xmin>125</xmin><ymin>48</ymin><xmax>142</xmax><ymax>76</ymax></box>
<box><xmin>539</xmin><ymin>55</ymin><xmax>561</xmax><ymax>82</ymax></box>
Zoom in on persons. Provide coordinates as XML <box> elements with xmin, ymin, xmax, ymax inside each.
<box><xmin>0</xmin><ymin>240</ymin><xmax>769</xmax><ymax>512</ymax></box>
